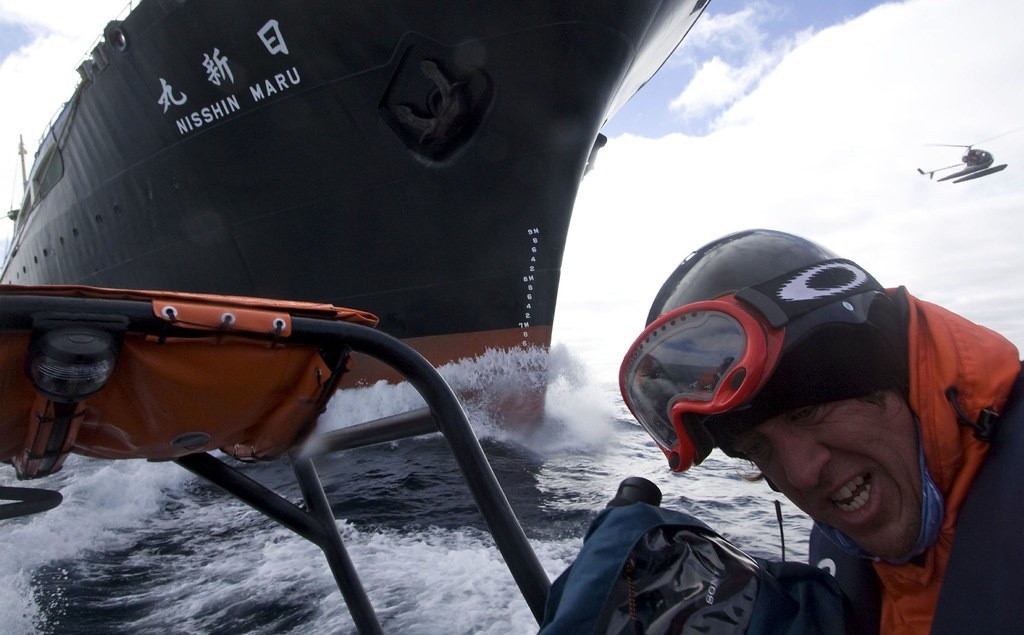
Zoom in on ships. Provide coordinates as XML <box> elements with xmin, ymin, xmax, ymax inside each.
<box><xmin>2</xmin><ymin>0</ymin><xmax>710</xmax><ymax>451</ymax></box>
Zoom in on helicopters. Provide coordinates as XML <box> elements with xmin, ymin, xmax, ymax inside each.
<box><xmin>913</xmin><ymin>127</ymin><xmax>1017</xmax><ymax>185</ymax></box>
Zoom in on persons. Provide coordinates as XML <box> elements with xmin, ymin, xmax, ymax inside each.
<box><xmin>620</xmin><ymin>230</ymin><xmax>1024</xmax><ymax>635</ymax></box>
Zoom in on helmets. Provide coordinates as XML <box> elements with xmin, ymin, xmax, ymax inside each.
<box><xmin>645</xmin><ymin>229</ymin><xmax>895</xmax><ymax>356</ymax></box>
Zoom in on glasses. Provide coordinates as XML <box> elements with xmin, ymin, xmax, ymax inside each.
<box><xmin>618</xmin><ymin>258</ymin><xmax>893</xmax><ymax>468</ymax></box>
